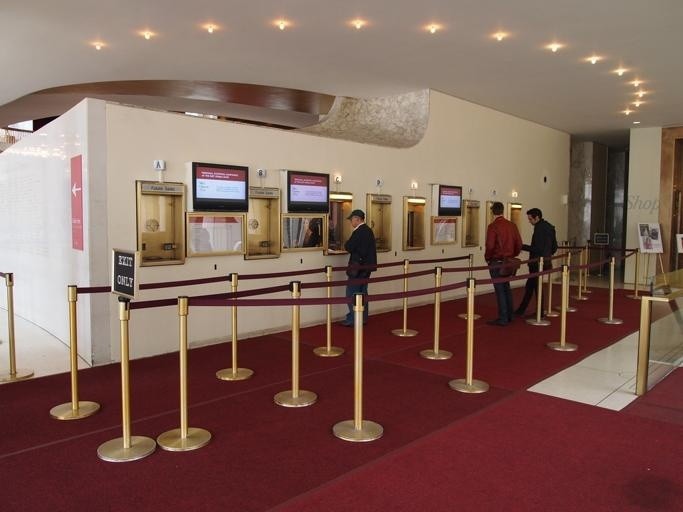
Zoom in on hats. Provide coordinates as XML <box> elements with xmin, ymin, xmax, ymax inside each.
<box><xmin>490</xmin><ymin>202</ymin><xmax>504</xmax><ymax>209</ymax></box>
<box><xmin>346</xmin><ymin>210</ymin><xmax>365</xmax><ymax>220</ymax></box>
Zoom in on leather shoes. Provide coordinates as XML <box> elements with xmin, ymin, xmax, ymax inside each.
<box><xmin>340</xmin><ymin>321</ymin><xmax>354</xmax><ymax>326</ymax></box>
<box><xmin>487</xmin><ymin>310</ymin><xmax>524</xmax><ymax>325</ymax></box>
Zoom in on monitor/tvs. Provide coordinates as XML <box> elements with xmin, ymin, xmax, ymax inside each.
<box><xmin>192</xmin><ymin>162</ymin><xmax>248</xmax><ymax>212</ymax></box>
<box><xmin>287</xmin><ymin>170</ymin><xmax>329</xmax><ymax>213</ymax></box>
<box><xmin>438</xmin><ymin>184</ymin><xmax>462</xmax><ymax>216</ymax></box>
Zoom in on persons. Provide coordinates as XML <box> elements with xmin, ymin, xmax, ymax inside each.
<box><xmin>308</xmin><ymin>218</ymin><xmax>337</xmax><ymax>251</ymax></box>
<box><xmin>509</xmin><ymin>207</ymin><xmax>558</xmax><ymax>320</ymax></box>
<box><xmin>479</xmin><ymin>201</ymin><xmax>522</xmax><ymax>327</ymax></box>
<box><xmin>340</xmin><ymin>209</ymin><xmax>377</xmax><ymax>327</ymax></box>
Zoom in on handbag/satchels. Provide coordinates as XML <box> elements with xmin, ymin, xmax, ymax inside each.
<box><xmin>346</xmin><ymin>262</ymin><xmax>361</xmax><ymax>277</ymax></box>
<box><xmin>499</xmin><ymin>257</ymin><xmax>521</xmax><ymax>275</ymax></box>
